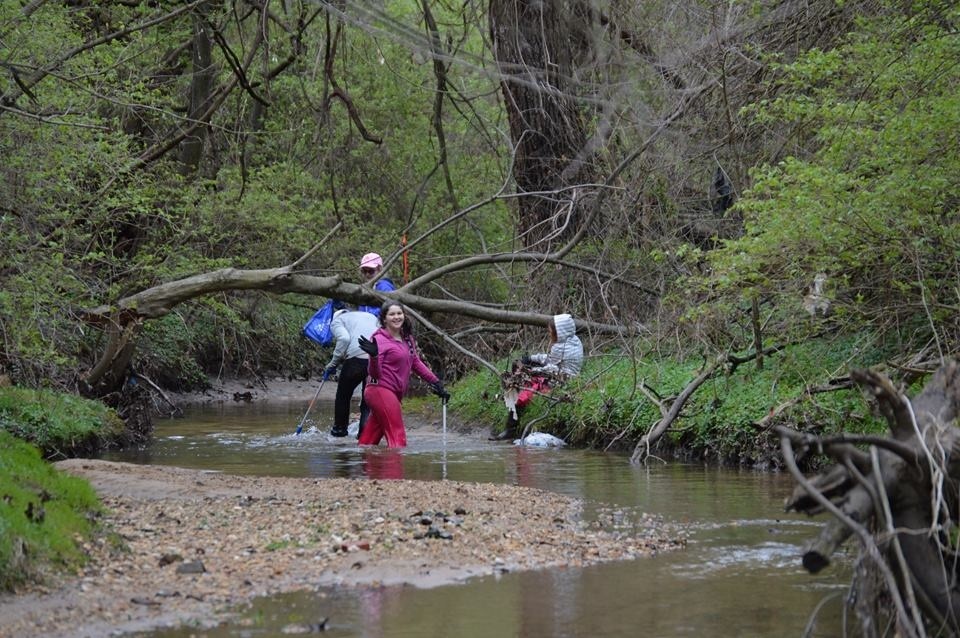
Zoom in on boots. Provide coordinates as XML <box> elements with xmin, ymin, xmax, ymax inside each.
<box><xmin>488</xmin><ymin>404</ymin><xmax>525</xmax><ymax>440</ymax></box>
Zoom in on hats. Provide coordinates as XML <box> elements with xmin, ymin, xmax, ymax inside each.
<box><xmin>359</xmin><ymin>253</ymin><xmax>383</xmax><ymax>268</ymax></box>
<box><xmin>333</xmin><ymin>309</ymin><xmax>348</xmax><ymax>319</ymax></box>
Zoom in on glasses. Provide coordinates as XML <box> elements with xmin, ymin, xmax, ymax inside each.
<box><xmin>361</xmin><ymin>269</ymin><xmax>378</xmax><ymax>275</ymax></box>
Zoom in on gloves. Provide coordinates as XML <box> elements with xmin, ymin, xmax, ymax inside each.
<box><xmin>435</xmin><ymin>382</ymin><xmax>447</xmax><ymax>398</ymax></box>
<box><xmin>325</xmin><ymin>363</ymin><xmax>336</xmax><ymax>374</ymax></box>
<box><xmin>522</xmin><ymin>357</ymin><xmax>529</xmax><ymax>365</ymax></box>
<box><xmin>358</xmin><ymin>335</ymin><xmax>379</xmax><ymax>357</ymax></box>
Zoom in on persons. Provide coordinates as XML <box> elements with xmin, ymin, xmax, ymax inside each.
<box><xmin>322</xmin><ymin>309</ymin><xmax>381</xmax><ymax>440</ymax></box>
<box><xmin>360</xmin><ymin>253</ymin><xmax>394</xmax><ymax>317</ymax></box>
<box><xmin>358</xmin><ymin>299</ymin><xmax>450</xmax><ymax>448</ymax></box>
<box><xmin>489</xmin><ymin>313</ymin><xmax>584</xmax><ymax>441</ymax></box>
